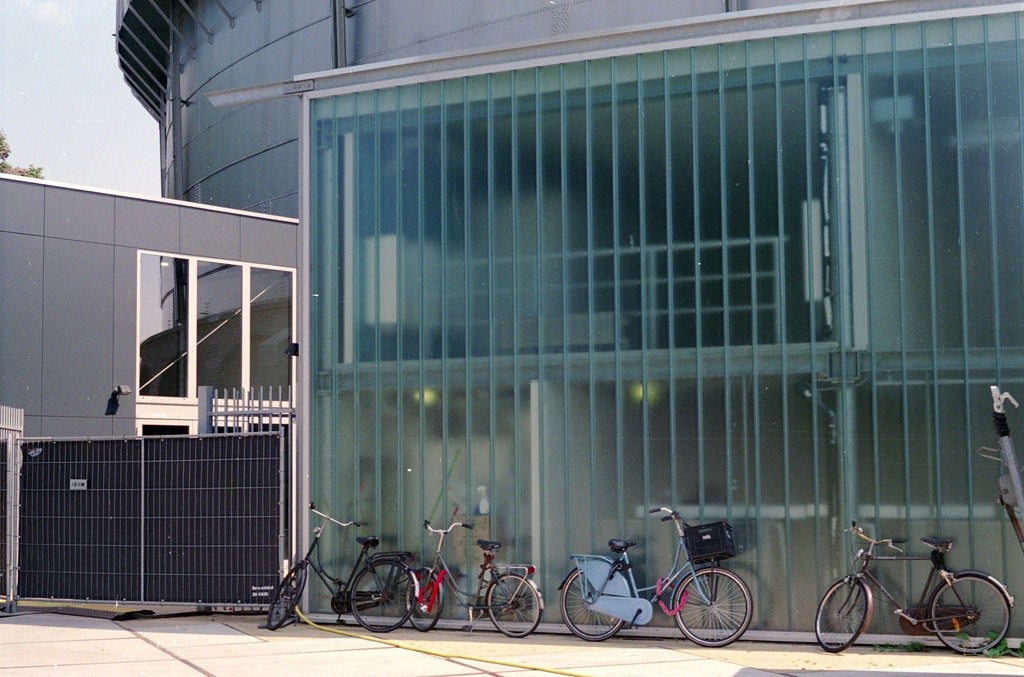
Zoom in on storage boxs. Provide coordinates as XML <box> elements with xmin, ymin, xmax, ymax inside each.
<box><xmin>452</xmin><ymin>515</ymin><xmax>504</xmax><ymax>565</ymax></box>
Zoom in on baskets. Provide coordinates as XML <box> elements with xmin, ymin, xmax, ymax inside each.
<box><xmin>685</xmin><ymin>522</ymin><xmax>736</xmax><ymax>563</ymax></box>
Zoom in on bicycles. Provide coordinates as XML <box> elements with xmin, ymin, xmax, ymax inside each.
<box><xmin>814</xmin><ymin>518</ymin><xmax>1016</xmax><ymax>656</ymax></box>
<box><xmin>404</xmin><ymin>519</ymin><xmax>545</xmax><ymax>642</ymax></box>
<box><xmin>267</xmin><ymin>502</ymin><xmax>420</xmax><ymax>634</ymax></box>
<box><xmin>557</xmin><ymin>505</ymin><xmax>752</xmax><ymax>650</ymax></box>
